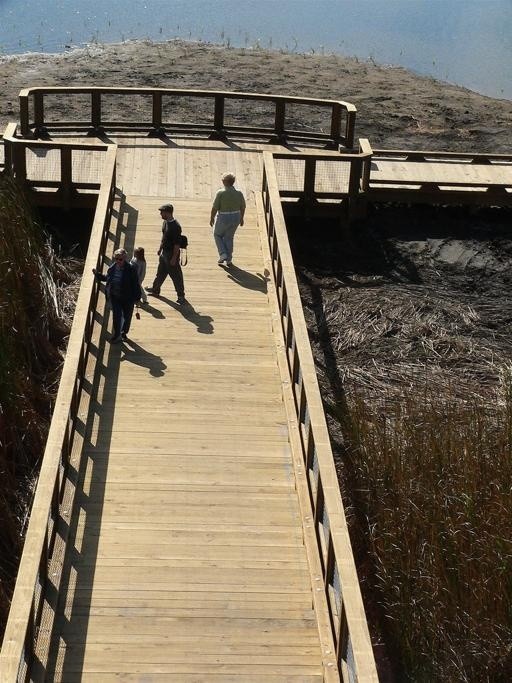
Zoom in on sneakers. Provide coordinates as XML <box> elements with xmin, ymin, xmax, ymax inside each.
<box><xmin>145</xmin><ymin>287</ymin><xmax>160</xmax><ymax>295</ymax></box>
<box><xmin>135</xmin><ymin>295</ymin><xmax>147</xmax><ymax>308</ymax></box>
<box><xmin>218</xmin><ymin>252</ymin><xmax>232</xmax><ymax>266</ymax></box>
<box><xmin>177</xmin><ymin>296</ymin><xmax>184</xmax><ymax>303</ymax></box>
<box><xmin>111</xmin><ymin>331</ymin><xmax>129</xmax><ymax>341</ymax></box>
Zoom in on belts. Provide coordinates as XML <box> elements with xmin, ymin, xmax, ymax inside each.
<box><xmin>217</xmin><ymin>210</ymin><xmax>241</xmax><ymax>214</ymax></box>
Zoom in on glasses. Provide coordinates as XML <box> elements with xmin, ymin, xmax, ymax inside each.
<box><xmin>116</xmin><ymin>257</ymin><xmax>123</xmax><ymax>261</ymax></box>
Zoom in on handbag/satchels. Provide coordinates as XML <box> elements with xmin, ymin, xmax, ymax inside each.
<box><xmin>180</xmin><ymin>235</ymin><xmax>188</xmax><ymax>248</ymax></box>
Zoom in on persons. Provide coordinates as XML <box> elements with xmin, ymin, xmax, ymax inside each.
<box><xmin>210</xmin><ymin>171</ymin><xmax>246</xmax><ymax>265</ymax></box>
<box><xmin>130</xmin><ymin>246</ymin><xmax>151</xmax><ymax>304</ymax></box>
<box><xmin>145</xmin><ymin>202</ymin><xmax>187</xmax><ymax>306</ymax></box>
<box><xmin>105</xmin><ymin>247</ymin><xmax>141</xmax><ymax>342</ymax></box>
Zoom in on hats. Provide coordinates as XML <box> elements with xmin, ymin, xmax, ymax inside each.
<box><xmin>159</xmin><ymin>203</ymin><xmax>174</xmax><ymax>211</ymax></box>
